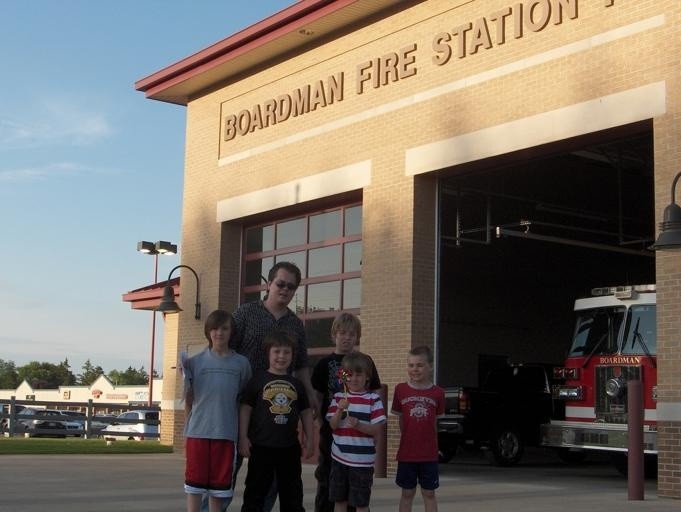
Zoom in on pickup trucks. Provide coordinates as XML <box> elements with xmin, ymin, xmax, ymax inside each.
<box><xmin>437</xmin><ymin>361</ymin><xmax>590</xmax><ymax>467</ymax></box>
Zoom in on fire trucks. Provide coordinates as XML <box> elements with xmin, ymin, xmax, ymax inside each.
<box><xmin>540</xmin><ymin>284</ymin><xmax>657</xmax><ymax>480</ymax></box>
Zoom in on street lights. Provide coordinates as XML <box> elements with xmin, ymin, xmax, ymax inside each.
<box><xmin>137</xmin><ymin>240</ymin><xmax>178</xmax><ymax>408</ymax></box>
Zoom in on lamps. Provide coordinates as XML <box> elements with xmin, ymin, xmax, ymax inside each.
<box><xmin>154</xmin><ymin>265</ymin><xmax>201</xmax><ymax>323</ymax></box>
<box><xmin>647</xmin><ymin>168</ymin><xmax>681</xmax><ymax>253</ymax></box>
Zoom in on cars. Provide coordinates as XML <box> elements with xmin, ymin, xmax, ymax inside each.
<box><xmin>0</xmin><ymin>405</ymin><xmax>118</xmax><ymax>438</ymax></box>
<box><xmin>100</xmin><ymin>410</ymin><xmax>159</xmax><ymax>441</ymax></box>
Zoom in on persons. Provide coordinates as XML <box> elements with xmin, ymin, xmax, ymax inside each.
<box><xmin>183</xmin><ymin>309</ymin><xmax>252</xmax><ymax>512</ymax></box>
<box><xmin>391</xmin><ymin>343</ymin><xmax>445</xmax><ymax>512</ymax></box>
<box><xmin>310</xmin><ymin>311</ymin><xmax>382</xmax><ymax>512</ymax></box>
<box><xmin>201</xmin><ymin>261</ymin><xmax>322</xmax><ymax>511</ymax></box>
<box><xmin>325</xmin><ymin>351</ymin><xmax>387</xmax><ymax>512</ymax></box>
<box><xmin>236</xmin><ymin>328</ymin><xmax>318</xmax><ymax>511</ymax></box>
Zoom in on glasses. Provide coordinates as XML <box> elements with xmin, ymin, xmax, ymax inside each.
<box><xmin>276</xmin><ymin>279</ymin><xmax>297</xmax><ymax>291</ymax></box>
